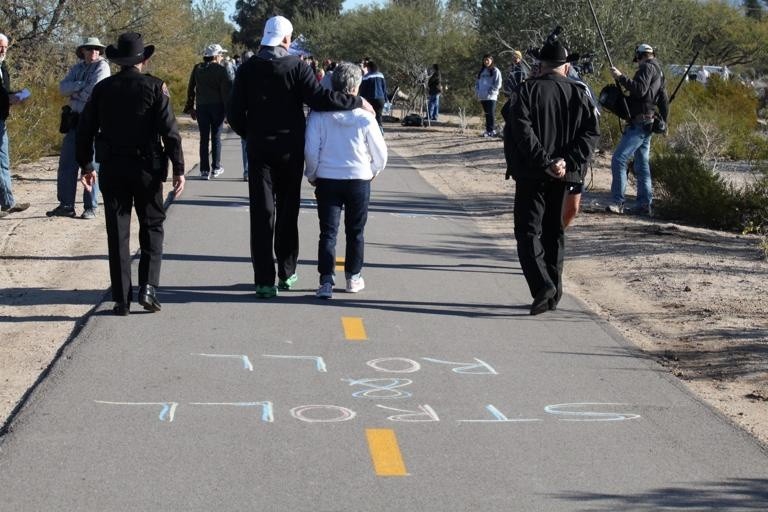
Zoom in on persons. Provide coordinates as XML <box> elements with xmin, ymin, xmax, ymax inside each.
<box><xmin>304</xmin><ymin>62</ymin><xmax>389</xmax><ymax>297</ymax></box>
<box><xmin>424</xmin><ymin>63</ymin><xmax>442</xmax><ymax>120</ymax></box>
<box><xmin>696</xmin><ymin>64</ymin><xmax>710</xmax><ymax>82</ymax></box>
<box><xmin>74</xmin><ymin>32</ymin><xmax>186</xmax><ymax>314</ymax></box>
<box><xmin>719</xmin><ymin>66</ymin><xmax>729</xmax><ymax>81</ymax></box>
<box><xmin>475</xmin><ymin>50</ymin><xmax>601</xmax><ymax>137</ymax></box>
<box><xmin>500</xmin><ymin>39</ymin><xmax>601</xmax><ymax>314</ymax></box>
<box><xmin>0</xmin><ymin>34</ymin><xmax>29</xmax><ymax>218</ymax></box>
<box><xmin>182</xmin><ymin>43</ymin><xmax>232</xmax><ymax>181</ymax></box>
<box><xmin>225</xmin><ymin>14</ymin><xmax>376</xmax><ymax>298</ymax></box>
<box><xmin>606</xmin><ymin>42</ymin><xmax>671</xmax><ymax>216</ymax></box>
<box><xmin>45</xmin><ymin>36</ymin><xmax>111</xmax><ymax>219</ymax></box>
<box><xmin>241</xmin><ymin>139</ymin><xmax>249</xmax><ymax>182</ymax></box>
<box><xmin>683</xmin><ymin>68</ymin><xmax>690</xmax><ymax>81</ymax></box>
<box><xmin>220</xmin><ymin>53</ymin><xmax>389</xmax><ymax>136</ymax></box>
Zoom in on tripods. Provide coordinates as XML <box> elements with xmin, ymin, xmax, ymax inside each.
<box><xmin>405</xmin><ymin>83</ymin><xmax>431</xmax><ymax>128</ymax></box>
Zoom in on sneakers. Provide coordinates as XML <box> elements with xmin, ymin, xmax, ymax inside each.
<box><xmin>483</xmin><ymin>130</ymin><xmax>498</xmax><ymax>138</ymax></box>
<box><xmin>199</xmin><ymin>167</ymin><xmax>224</xmax><ymax>180</ymax></box>
<box><xmin>0</xmin><ymin>203</ymin><xmax>30</xmax><ymax>217</ymax></box>
<box><xmin>606</xmin><ymin>202</ymin><xmax>652</xmax><ymax>215</ymax></box>
<box><xmin>256</xmin><ymin>272</ymin><xmax>364</xmax><ymax>298</ymax></box>
<box><xmin>46</xmin><ymin>203</ymin><xmax>96</xmax><ymax>219</ymax></box>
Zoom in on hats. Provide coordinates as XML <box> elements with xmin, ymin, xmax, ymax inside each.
<box><xmin>76</xmin><ymin>32</ymin><xmax>155</xmax><ymax>65</ymax></box>
<box><xmin>512</xmin><ymin>50</ymin><xmax>522</xmax><ymax>60</ymax></box>
<box><xmin>260</xmin><ymin>15</ymin><xmax>294</xmax><ymax>47</ymax></box>
<box><xmin>202</xmin><ymin>44</ymin><xmax>228</xmax><ymax>57</ymax></box>
<box><xmin>633</xmin><ymin>44</ymin><xmax>653</xmax><ymax>62</ymax></box>
<box><xmin>529</xmin><ymin>35</ymin><xmax>580</xmax><ymax>62</ymax></box>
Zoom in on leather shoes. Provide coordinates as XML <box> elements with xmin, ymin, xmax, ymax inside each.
<box><xmin>530</xmin><ymin>284</ymin><xmax>558</xmax><ymax>314</ymax></box>
<box><xmin>114</xmin><ymin>284</ymin><xmax>161</xmax><ymax>315</ymax></box>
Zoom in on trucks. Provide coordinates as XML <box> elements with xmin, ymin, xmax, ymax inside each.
<box><xmin>662</xmin><ymin>64</ymin><xmax>739</xmax><ymax>86</ymax></box>
